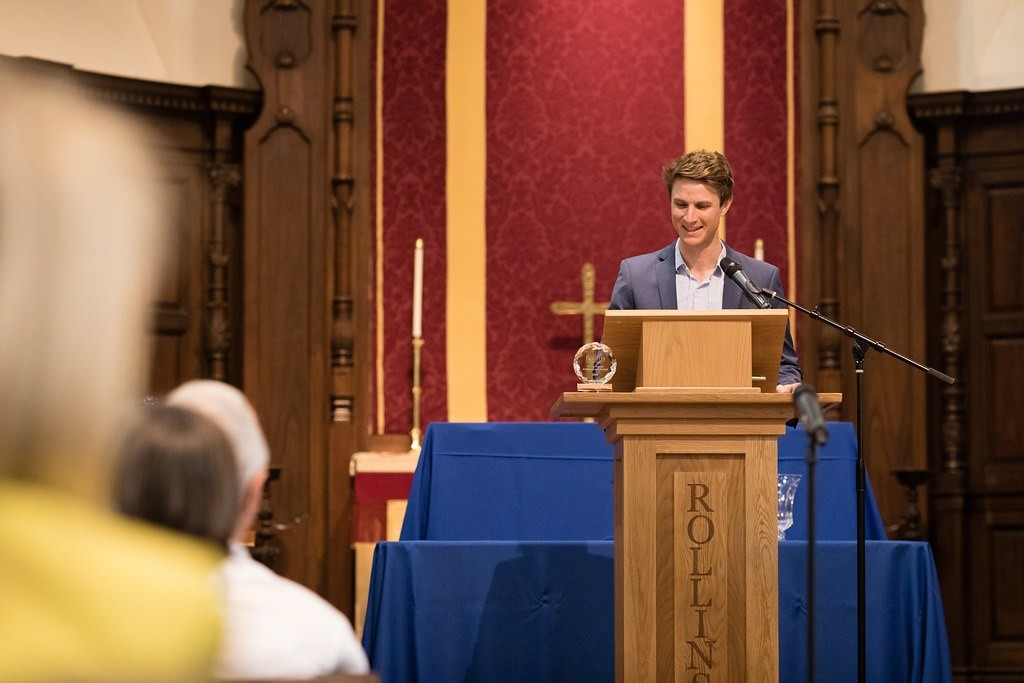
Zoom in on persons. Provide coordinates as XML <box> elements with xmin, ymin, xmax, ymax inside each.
<box><xmin>593</xmin><ymin>149</ymin><xmax>803</xmax><ymax>429</ymax></box>
<box><xmin>0</xmin><ymin>61</ymin><xmax>374</xmax><ymax>683</ymax></box>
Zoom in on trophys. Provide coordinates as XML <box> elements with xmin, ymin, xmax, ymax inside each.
<box><xmin>573</xmin><ymin>342</ymin><xmax>617</xmax><ymax>392</ymax></box>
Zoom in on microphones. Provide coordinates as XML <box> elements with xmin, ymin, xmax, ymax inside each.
<box><xmin>793</xmin><ymin>383</ymin><xmax>830</xmax><ymax>446</ymax></box>
<box><xmin>720</xmin><ymin>256</ymin><xmax>774</xmax><ymax>309</ymax></box>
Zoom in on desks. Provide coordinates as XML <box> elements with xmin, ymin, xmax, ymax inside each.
<box><xmin>347</xmin><ymin>452</ymin><xmax>421</xmax><ymax>642</ymax></box>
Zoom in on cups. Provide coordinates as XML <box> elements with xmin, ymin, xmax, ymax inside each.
<box><xmin>777</xmin><ymin>473</ymin><xmax>803</xmax><ymax>539</ymax></box>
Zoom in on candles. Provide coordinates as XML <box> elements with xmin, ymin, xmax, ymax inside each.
<box><xmin>411</xmin><ymin>238</ymin><xmax>424</xmax><ymax>339</ymax></box>
<box><xmin>754</xmin><ymin>238</ymin><xmax>764</xmax><ymax>262</ymax></box>
